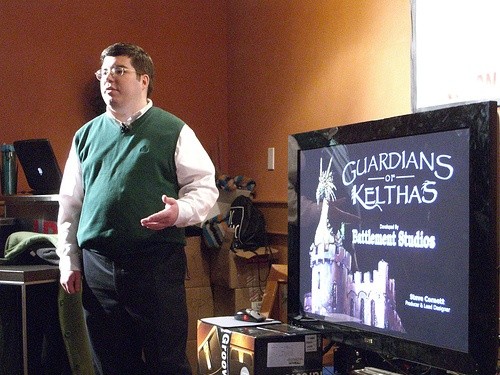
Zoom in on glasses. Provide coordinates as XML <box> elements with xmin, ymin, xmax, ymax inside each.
<box><xmin>95</xmin><ymin>67</ymin><xmax>148</xmax><ymax>81</ymax></box>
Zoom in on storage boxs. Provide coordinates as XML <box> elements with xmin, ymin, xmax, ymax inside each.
<box><xmin>185</xmin><ymin>228</ymin><xmax>335</xmax><ymax>375</ymax></box>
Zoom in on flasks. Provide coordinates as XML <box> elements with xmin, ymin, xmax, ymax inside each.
<box><xmin>0</xmin><ymin>143</ymin><xmax>19</xmax><ymax>196</ymax></box>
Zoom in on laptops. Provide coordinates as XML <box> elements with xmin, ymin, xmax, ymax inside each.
<box><xmin>13</xmin><ymin>138</ymin><xmax>63</xmax><ymax>194</ymax></box>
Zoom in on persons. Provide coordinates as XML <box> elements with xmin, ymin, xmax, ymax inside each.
<box><xmin>58</xmin><ymin>41</ymin><xmax>221</xmax><ymax>375</ymax></box>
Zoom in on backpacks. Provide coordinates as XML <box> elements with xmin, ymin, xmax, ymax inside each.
<box><xmin>226</xmin><ymin>195</ymin><xmax>267</xmax><ymax>250</ymax></box>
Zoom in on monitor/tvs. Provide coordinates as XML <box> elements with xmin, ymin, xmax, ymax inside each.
<box><xmin>286</xmin><ymin>101</ymin><xmax>500</xmax><ymax>375</ymax></box>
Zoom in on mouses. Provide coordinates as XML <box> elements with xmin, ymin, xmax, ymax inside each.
<box><xmin>234</xmin><ymin>308</ymin><xmax>267</xmax><ymax>323</ymax></box>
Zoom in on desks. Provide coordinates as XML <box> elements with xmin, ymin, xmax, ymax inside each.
<box><xmin>0</xmin><ymin>265</ymin><xmax>59</xmax><ymax>375</ymax></box>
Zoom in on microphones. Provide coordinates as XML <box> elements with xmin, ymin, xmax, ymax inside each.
<box><xmin>121</xmin><ymin>123</ymin><xmax>131</xmax><ymax>133</ymax></box>
